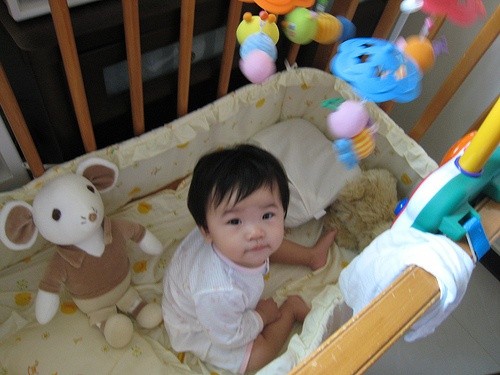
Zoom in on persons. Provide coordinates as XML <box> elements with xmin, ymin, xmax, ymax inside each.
<box><xmin>162</xmin><ymin>144</ymin><xmax>338</xmax><ymax>375</ymax></box>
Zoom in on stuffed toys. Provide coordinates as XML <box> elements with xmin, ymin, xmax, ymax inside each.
<box><xmin>239</xmin><ymin>0</ymin><xmax>326</xmax><ymax>14</ymax></box>
<box><xmin>399</xmin><ymin>0</ymin><xmax>485</xmax><ymax>27</ymax></box>
<box><xmin>323</xmin><ymin>91</ymin><xmax>380</xmax><ymax>172</ymax></box>
<box><xmin>236</xmin><ymin>10</ymin><xmax>280</xmax><ymax>85</ymax></box>
<box><xmin>1</xmin><ymin>158</ymin><xmax>165</xmax><ymax>347</ymax></box>
<box><xmin>325</xmin><ymin>168</ymin><xmax>406</xmax><ymax>251</ymax></box>
<box><xmin>385</xmin><ymin>29</ymin><xmax>437</xmax><ymax>112</ymax></box>
<box><xmin>282</xmin><ymin>6</ymin><xmax>356</xmax><ymax>45</ymax></box>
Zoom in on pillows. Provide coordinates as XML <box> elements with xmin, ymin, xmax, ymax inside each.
<box><xmin>246</xmin><ymin>117</ymin><xmax>364</xmax><ymax>230</ymax></box>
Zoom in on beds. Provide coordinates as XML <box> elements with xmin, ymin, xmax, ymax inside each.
<box><xmin>0</xmin><ymin>0</ymin><xmax>500</xmax><ymax>375</ymax></box>
